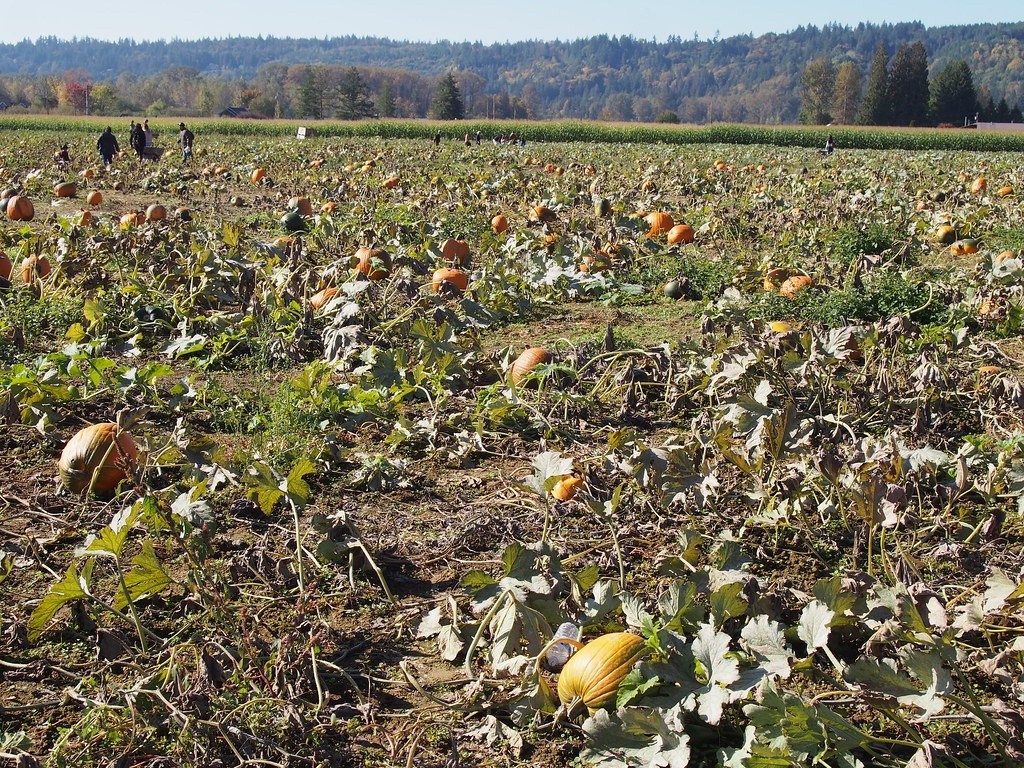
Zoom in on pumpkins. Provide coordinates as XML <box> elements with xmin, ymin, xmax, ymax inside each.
<box><xmin>557</xmin><ymin>633</ymin><xmax>649</xmax><ymax>726</ymax></box>
<box><xmin>0</xmin><ymin>148</ymin><xmax>1018</xmax><ymax>499</ymax></box>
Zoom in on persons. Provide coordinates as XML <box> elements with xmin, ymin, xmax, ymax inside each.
<box><xmin>130</xmin><ymin>119</ymin><xmax>152</xmax><ymax>161</ymax></box>
<box><xmin>826</xmin><ymin>135</ymin><xmax>833</xmax><ymax>154</ymax></box>
<box><xmin>58</xmin><ymin>144</ymin><xmax>70</xmax><ymax>170</ymax></box>
<box><xmin>176</xmin><ymin>122</ymin><xmax>192</xmax><ymax>164</ymax></box>
<box><xmin>434</xmin><ymin>130</ymin><xmax>526</xmax><ymax>147</ymax></box>
<box><xmin>96</xmin><ymin>126</ymin><xmax>120</xmax><ymax>164</ymax></box>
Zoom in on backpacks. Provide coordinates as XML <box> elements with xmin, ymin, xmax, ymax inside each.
<box><xmin>132</xmin><ymin>130</ymin><xmax>141</xmax><ymax>148</ymax></box>
<box><xmin>54</xmin><ymin>151</ymin><xmax>62</xmax><ymax>161</ymax></box>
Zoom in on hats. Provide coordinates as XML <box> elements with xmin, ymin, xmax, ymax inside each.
<box><xmin>61</xmin><ymin>144</ymin><xmax>69</xmax><ymax>149</ymax></box>
<box><xmin>178</xmin><ymin>122</ymin><xmax>185</xmax><ymax>126</ymax></box>
